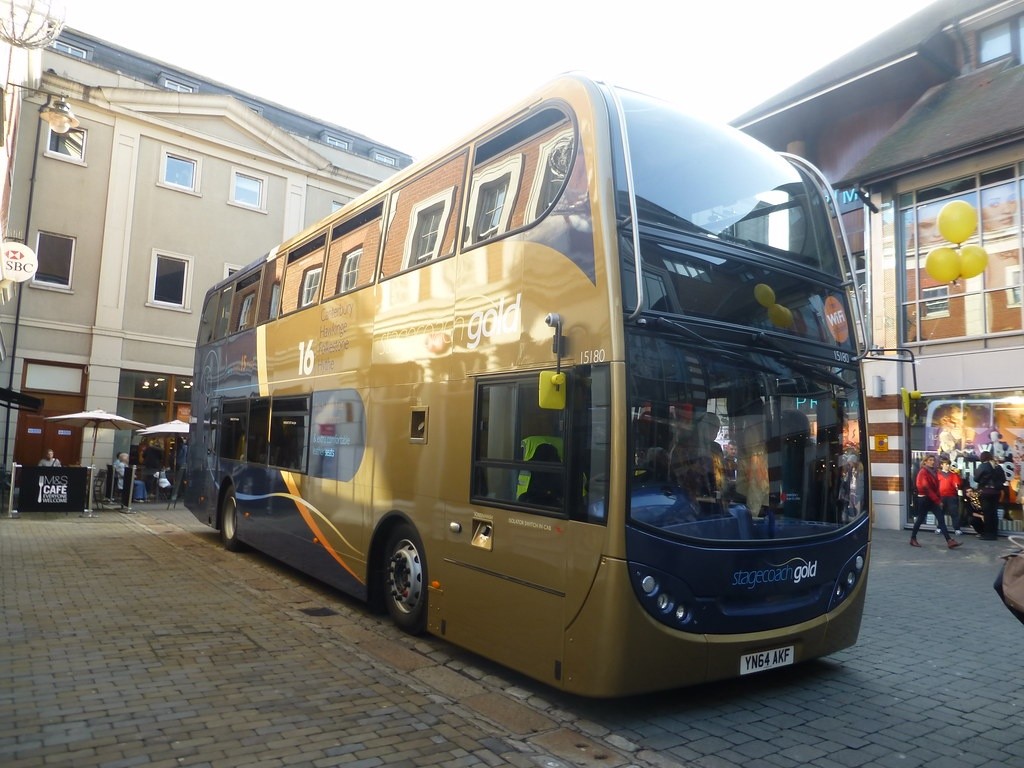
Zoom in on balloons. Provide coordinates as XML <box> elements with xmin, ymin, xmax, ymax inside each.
<box><xmin>938</xmin><ymin>200</ymin><xmax>978</xmax><ymax>244</ymax></box>
<box><xmin>753</xmin><ymin>283</ymin><xmax>793</xmax><ymax>327</ymax></box>
<box><xmin>925</xmin><ymin>248</ymin><xmax>962</xmax><ymax>283</ymax></box>
<box><xmin>959</xmin><ymin>246</ymin><xmax>988</xmax><ymax>280</ymax></box>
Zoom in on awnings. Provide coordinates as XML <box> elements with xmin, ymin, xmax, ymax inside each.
<box><xmin>0</xmin><ymin>387</ymin><xmax>45</xmax><ymax>416</ymax></box>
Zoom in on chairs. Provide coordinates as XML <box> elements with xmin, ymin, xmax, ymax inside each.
<box><xmin>93</xmin><ymin>464</ymin><xmax>125</xmax><ymax>510</ymax></box>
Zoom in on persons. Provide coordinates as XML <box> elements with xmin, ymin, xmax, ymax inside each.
<box><xmin>962</xmin><ymin>182</ymin><xmax>1020</xmax><ymax>289</ymax></box>
<box><xmin>934</xmin><ymin>405</ymin><xmax>977</xmax><ymax>452</ymax></box>
<box><xmin>984</xmin><ymin>431</ymin><xmax>1015</xmax><ymax>522</ymax></box>
<box><xmin>910</xmin><ymin>454</ymin><xmax>963</xmax><ymax>547</ymax></box>
<box><xmin>113</xmin><ymin>452</ymin><xmax>150</xmax><ymax>503</ymax></box>
<box><xmin>38</xmin><ymin>448</ymin><xmax>62</xmax><ymax>467</ymax></box>
<box><xmin>964</xmin><ymin>487</ymin><xmax>984</xmax><ymax>537</ymax></box>
<box><xmin>974</xmin><ymin>451</ymin><xmax>1006</xmax><ymax>541</ymax></box>
<box><xmin>169</xmin><ymin>435</ymin><xmax>188</xmax><ymax>481</ymax></box>
<box><xmin>143</xmin><ymin>441</ymin><xmax>165</xmax><ymax>493</ymax></box>
<box><xmin>635</xmin><ymin>407</ymin><xmax>815</xmax><ymax>518</ymax></box>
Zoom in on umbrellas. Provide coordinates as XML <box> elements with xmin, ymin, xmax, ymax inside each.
<box><xmin>42</xmin><ymin>409</ymin><xmax>144</xmax><ymax>468</ymax></box>
<box><xmin>136</xmin><ymin>420</ymin><xmax>190</xmax><ymax>470</ymax></box>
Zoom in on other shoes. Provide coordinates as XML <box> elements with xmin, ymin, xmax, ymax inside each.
<box><xmin>910</xmin><ymin>538</ymin><xmax>922</xmax><ymax>547</ymax></box>
<box><xmin>134</xmin><ymin>499</ymin><xmax>144</xmax><ymax>502</ymax></box>
<box><xmin>144</xmin><ymin>499</ymin><xmax>150</xmax><ymax>502</ymax></box>
<box><xmin>935</xmin><ymin>528</ymin><xmax>941</xmax><ymax>534</ymax></box>
<box><xmin>976</xmin><ymin>533</ymin><xmax>982</xmax><ymax>537</ymax></box>
<box><xmin>955</xmin><ymin>530</ymin><xmax>962</xmax><ymax>536</ymax></box>
<box><xmin>150</xmin><ymin>494</ymin><xmax>155</xmax><ymax>498</ymax></box>
<box><xmin>1003</xmin><ymin>513</ymin><xmax>1014</xmax><ymax>521</ymax></box>
<box><xmin>948</xmin><ymin>538</ymin><xmax>963</xmax><ymax>548</ymax></box>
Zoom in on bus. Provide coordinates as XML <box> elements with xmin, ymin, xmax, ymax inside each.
<box><xmin>182</xmin><ymin>68</ymin><xmax>926</xmax><ymax>704</ymax></box>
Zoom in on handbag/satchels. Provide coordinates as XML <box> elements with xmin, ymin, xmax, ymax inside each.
<box><xmin>999</xmin><ymin>536</ymin><xmax>1024</xmax><ymax>612</ymax></box>
